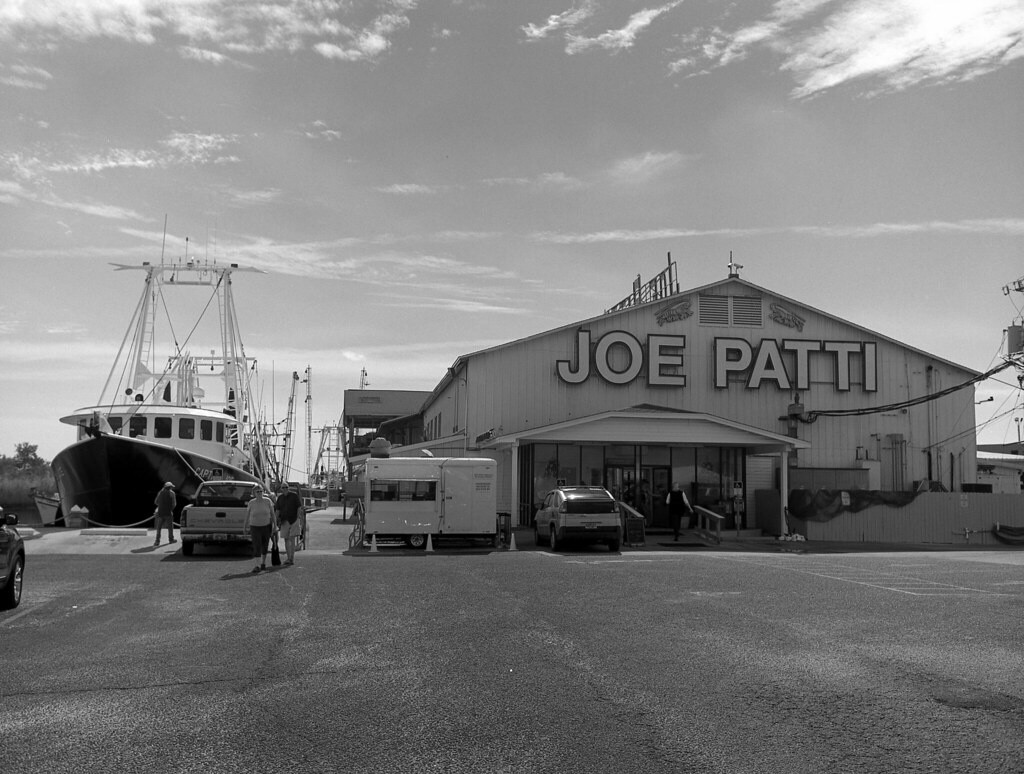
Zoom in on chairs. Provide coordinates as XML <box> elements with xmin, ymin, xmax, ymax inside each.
<box><xmin>240</xmin><ymin>492</ymin><xmax>252</xmax><ymax>502</ymax></box>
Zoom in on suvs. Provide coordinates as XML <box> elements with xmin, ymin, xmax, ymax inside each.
<box><xmin>533</xmin><ymin>485</ymin><xmax>622</xmax><ymax>551</ymax></box>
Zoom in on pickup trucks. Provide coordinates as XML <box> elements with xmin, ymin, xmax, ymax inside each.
<box><xmin>180</xmin><ymin>480</ymin><xmax>270</xmax><ymax>557</ymax></box>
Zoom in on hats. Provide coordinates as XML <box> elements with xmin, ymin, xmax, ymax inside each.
<box><xmin>164</xmin><ymin>482</ymin><xmax>175</xmax><ymax>488</ymax></box>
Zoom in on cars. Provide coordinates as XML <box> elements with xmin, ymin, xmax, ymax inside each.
<box><xmin>0</xmin><ymin>505</ymin><xmax>25</xmax><ymax>608</ymax></box>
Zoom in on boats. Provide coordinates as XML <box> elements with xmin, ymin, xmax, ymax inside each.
<box><xmin>50</xmin><ymin>209</ymin><xmax>350</xmax><ymax>530</ymax></box>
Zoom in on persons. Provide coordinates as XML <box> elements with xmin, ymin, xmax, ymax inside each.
<box><xmin>665</xmin><ymin>482</ymin><xmax>694</xmax><ymax>542</ymax></box>
<box><xmin>635</xmin><ymin>479</ymin><xmax>662</xmax><ymax>528</ymax></box>
<box><xmin>155</xmin><ymin>482</ymin><xmax>177</xmax><ymax>545</ymax></box>
<box><xmin>274</xmin><ymin>482</ymin><xmax>301</xmax><ymax>565</ymax></box>
<box><xmin>243</xmin><ymin>486</ymin><xmax>277</xmax><ymax>573</ymax></box>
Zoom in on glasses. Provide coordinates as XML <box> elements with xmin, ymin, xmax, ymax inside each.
<box><xmin>256</xmin><ymin>491</ymin><xmax>262</xmax><ymax>493</ymax></box>
<box><xmin>282</xmin><ymin>486</ymin><xmax>288</xmax><ymax>489</ymax></box>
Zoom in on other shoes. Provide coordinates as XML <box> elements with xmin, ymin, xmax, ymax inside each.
<box><xmin>154</xmin><ymin>543</ymin><xmax>160</xmax><ymax>547</ymax></box>
<box><xmin>250</xmin><ymin>566</ymin><xmax>260</xmax><ymax>573</ymax></box>
<box><xmin>169</xmin><ymin>539</ymin><xmax>177</xmax><ymax>544</ymax></box>
<box><xmin>260</xmin><ymin>564</ymin><xmax>266</xmax><ymax>571</ymax></box>
<box><xmin>285</xmin><ymin>559</ymin><xmax>294</xmax><ymax>565</ymax></box>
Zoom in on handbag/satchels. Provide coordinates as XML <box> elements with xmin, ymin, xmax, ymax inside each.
<box><xmin>271</xmin><ymin>536</ymin><xmax>281</xmax><ymax>566</ymax></box>
